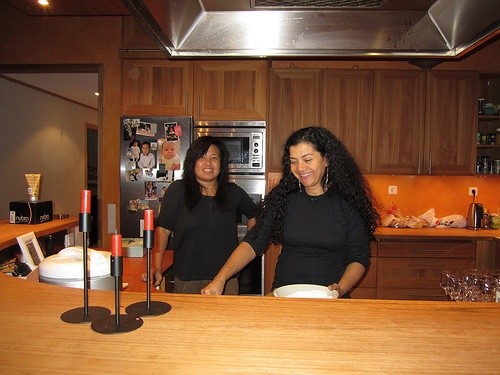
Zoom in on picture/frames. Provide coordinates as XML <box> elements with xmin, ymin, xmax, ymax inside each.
<box><xmin>17</xmin><ymin>231</ymin><xmax>46</xmax><ymax>270</ymax></box>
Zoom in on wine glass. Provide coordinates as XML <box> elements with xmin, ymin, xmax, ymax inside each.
<box><xmin>439</xmin><ymin>269</ymin><xmax>500</xmax><ymax>302</ymax></box>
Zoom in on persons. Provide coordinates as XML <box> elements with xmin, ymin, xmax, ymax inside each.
<box><xmin>201</xmin><ymin>126</ymin><xmax>381</xmax><ymax>298</ymax></box>
<box><xmin>141</xmin><ymin>136</ymin><xmax>256</xmax><ymax>295</ymax></box>
<box><xmin>147</xmin><ymin>182</ymin><xmax>153</xmax><ymax>193</ymax></box>
<box><xmin>137</xmin><ymin>141</ymin><xmax>156</xmax><ymax>168</ymax></box>
<box><xmin>167</xmin><ymin>128</ymin><xmax>177</xmax><ymax>138</ymax></box>
<box><xmin>159</xmin><ymin>141</ymin><xmax>180</xmax><ymax>170</ymax></box>
<box><xmin>161</xmin><ymin>187</ymin><xmax>166</xmax><ymax>196</ymax></box>
<box><xmin>131</xmin><ymin>142</ymin><xmax>140</xmax><ymax>161</ymax></box>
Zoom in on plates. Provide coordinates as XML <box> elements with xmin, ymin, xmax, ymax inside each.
<box><xmin>274</xmin><ymin>284</ymin><xmax>340</xmax><ymax>299</ymax></box>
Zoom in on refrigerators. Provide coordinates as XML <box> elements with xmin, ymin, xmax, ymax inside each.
<box><xmin>119</xmin><ymin>113</ymin><xmax>193</xmax><ymax>250</ymax></box>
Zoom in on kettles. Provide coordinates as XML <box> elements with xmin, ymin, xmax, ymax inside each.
<box><xmin>466</xmin><ymin>202</ymin><xmax>483</xmax><ymax>229</ymax></box>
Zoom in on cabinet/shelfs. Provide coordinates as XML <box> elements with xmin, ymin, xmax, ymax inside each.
<box><xmin>120</xmin><ymin>58</ymin><xmax>500</xmax><ymax>179</ymax></box>
<box><xmin>264</xmin><ymin>235</ymin><xmax>500</xmax><ymax>302</ymax></box>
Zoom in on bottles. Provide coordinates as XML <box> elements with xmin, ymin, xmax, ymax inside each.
<box><xmin>474</xmin><ymin>99</ymin><xmax>500</xmax><ymax>174</ymax></box>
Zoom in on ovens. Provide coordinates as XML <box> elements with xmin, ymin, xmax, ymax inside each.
<box><xmin>193</xmin><ymin>120</ymin><xmax>267</xmax><ymax>244</ymax></box>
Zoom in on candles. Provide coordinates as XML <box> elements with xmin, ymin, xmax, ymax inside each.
<box><xmin>80</xmin><ymin>187</ymin><xmax>92</xmax><ymax>213</ymax></box>
<box><xmin>111</xmin><ymin>229</ymin><xmax>123</xmax><ymax>256</ymax></box>
<box><xmin>144</xmin><ymin>207</ymin><xmax>154</xmax><ymax>230</ymax></box>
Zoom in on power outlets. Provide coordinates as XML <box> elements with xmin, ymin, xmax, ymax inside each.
<box><xmin>388</xmin><ymin>186</ymin><xmax>398</xmax><ymax>195</ymax></box>
<box><xmin>468</xmin><ymin>186</ymin><xmax>478</xmax><ymax>197</ymax></box>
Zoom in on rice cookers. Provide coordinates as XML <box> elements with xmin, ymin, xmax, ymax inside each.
<box><xmin>38</xmin><ymin>245</ymin><xmax>116</xmax><ymax>292</ymax></box>
